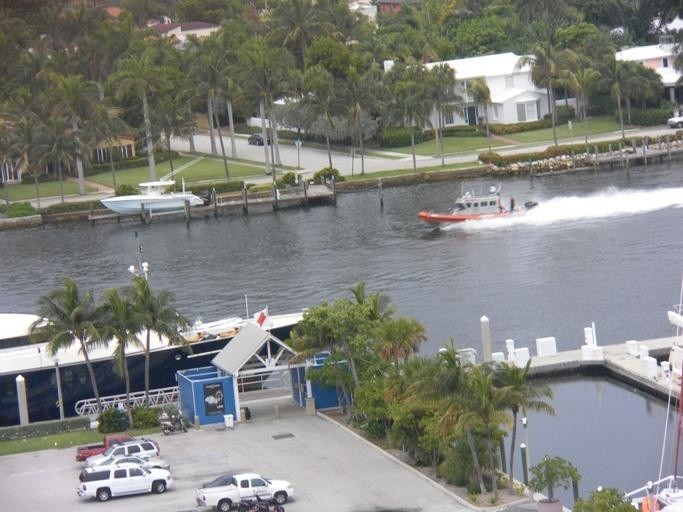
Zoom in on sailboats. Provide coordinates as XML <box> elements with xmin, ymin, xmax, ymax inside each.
<box><xmin>623</xmin><ymin>292</ymin><xmax>683</xmax><ymax>511</ymax></box>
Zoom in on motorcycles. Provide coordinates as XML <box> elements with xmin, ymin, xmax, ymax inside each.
<box><xmin>161</xmin><ymin>415</ymin><xmax>187</xmax><ymax>435</ymax></box>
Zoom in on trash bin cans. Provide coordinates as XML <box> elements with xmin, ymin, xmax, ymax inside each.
<box><xmin>223</xmin><ymin>414</ymin><xmax>234</xmax><ymax>427</ymax></box>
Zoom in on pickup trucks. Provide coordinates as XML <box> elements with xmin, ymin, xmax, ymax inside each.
<box><xmin>196</xmin><ymin>472</ymin><xmax>294</xmax><ymax>511</ymax></box>
<box><xmin>77</xmin><ymin>435</ymin><xmax>135</xmax><ymax>462</ymax></box>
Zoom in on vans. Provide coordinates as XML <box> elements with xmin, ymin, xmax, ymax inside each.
<box><xmin>75</xmin><ymin>439</ymin><xmax>173</xmax><ymax>501</ymax></box>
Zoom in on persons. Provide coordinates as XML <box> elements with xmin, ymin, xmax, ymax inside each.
<box><xmin>178</xmin><ymin>413</ymin><xmax>188</xmax><ymax>433</ymax></box>
<box><xmin>510</xmin><ymin>197</ymin><xmax>515</xmax><ymax>211</ymax></box>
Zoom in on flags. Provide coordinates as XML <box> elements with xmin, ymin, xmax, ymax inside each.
<box><xmin>253</xmin><ymin>307</ymin><xmax>269</xmax><ymax>330</ymax></box>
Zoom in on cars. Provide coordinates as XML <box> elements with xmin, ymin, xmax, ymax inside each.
<box><xmin>248</xmin><ymin>134</ymin><xmax>270</xmax><ymax>146</ymax></box>
<box><xmin>669</xmin><ymin>114</ymin><xmax>683</xmax><ymax>128</ymax></box>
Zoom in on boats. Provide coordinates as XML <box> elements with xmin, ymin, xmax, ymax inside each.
<box><xmin>419</xmin><ymin>194</ymin><xmax>523</xmax><ymax>225</ymax></box>
<box><xmin>102</xmin><ymin>182</ymin><xmax>204</xmax><ymax>216</ymax></box>
